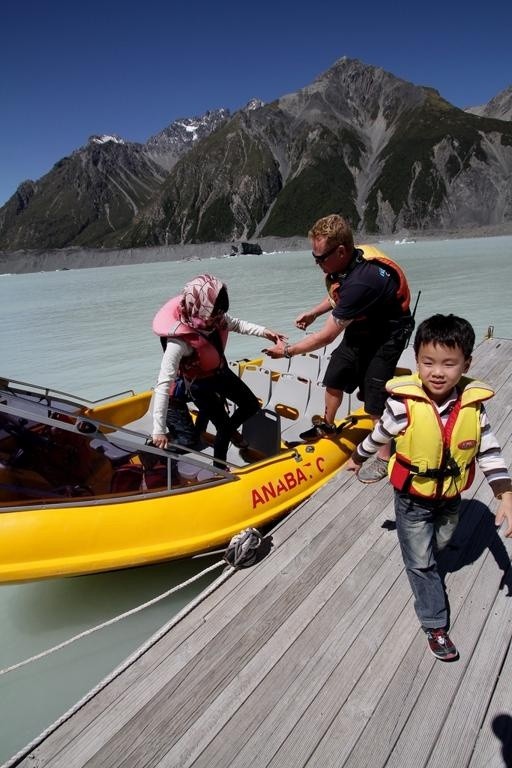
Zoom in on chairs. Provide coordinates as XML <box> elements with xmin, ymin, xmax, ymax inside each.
<box><xmin>103</xmin><ymin>330</ymin><xmax>418</xmax><ymax>485</ymax></box>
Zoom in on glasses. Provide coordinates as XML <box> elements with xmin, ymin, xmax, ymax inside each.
<box><xmin>312</xmin><ymin>245</ymin><xmax>338</xmax><ymax>260</ymax></box>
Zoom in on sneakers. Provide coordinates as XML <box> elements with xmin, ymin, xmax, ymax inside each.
<box><xmin>300</xmin><ymin>425</ymin><xmax>336</xmax><ymax>440</ymax></box>
<box><xmin>231</xmin><ymin>431</ymin><xmax>248</xmax><ymax>448</ymax></box>
<box><xmin>426</xmin><ymin>629</ymin><xmax>458</xmax><ymax>660</ymax></box>
<box><xmin>357</xmin><ymin>456</ymin><xmax>389</xmax><ymax>482</ymax></box>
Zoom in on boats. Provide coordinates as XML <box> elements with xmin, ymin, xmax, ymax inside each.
<box><xmin>0</xmin><ymin>356</ymin><xmax>414</xmax><ymax>587</ymax></box>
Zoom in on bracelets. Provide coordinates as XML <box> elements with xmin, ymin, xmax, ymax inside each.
<box><xmin>284</xmin><ymin>344</ymin><xmax>292</xmax><ymax>359</ymax></box>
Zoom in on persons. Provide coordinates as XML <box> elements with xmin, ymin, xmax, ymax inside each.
<box><xmin>262</xmin><ymin>214</ymin><xmax>416</xmax><ymax>483</ymax></box>
<box><xmin>344</xmin><ymin>312</ymin><xmax>512</xmax><ymax>659</ymax></box>
<box><xmin>151</xmin><ymin>273</ymin><xmax>289</xmax><ymax>474</ymax></box>
